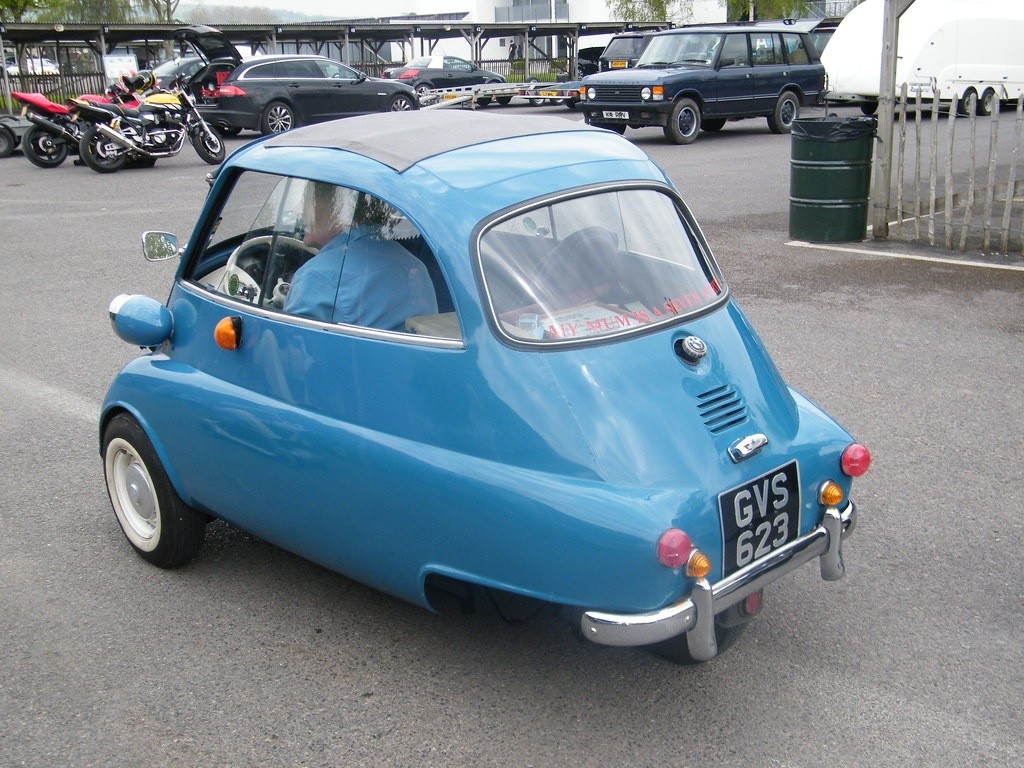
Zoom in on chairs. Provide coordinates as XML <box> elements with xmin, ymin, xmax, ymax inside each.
<box><xmin>689</xmin><ymin>42</ymin><xmax>704</xmax><ymax>53</ymax></box>
<box><xmin>519</xmin><ymin>226</ymin><xmax>621</xmax><ymax>307</ymax></box>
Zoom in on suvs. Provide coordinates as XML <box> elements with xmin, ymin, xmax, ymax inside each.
<box><xmin>580</xmin><ymin>16</ymin><xmax>847</xmax><ymax>145</ymax></box>
<box><xmin>599</xmin><ymin>29</ymin><xmax>716</xmax><ymax>76</ymax></box>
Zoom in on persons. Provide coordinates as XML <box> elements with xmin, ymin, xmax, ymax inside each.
<box><xmin>509</xmin><ymin>40</ymin><xmax>517</xmax><ymax>60</ymax></box>
<box><xmin>517</xmin><ymin>45</ymin><xmax>523</xmax><ymax>59</ymax></box>
<box><xmin>282</xmin><ymin>180</ymin><xmax>439</xmax><ymax>334</ymax></box>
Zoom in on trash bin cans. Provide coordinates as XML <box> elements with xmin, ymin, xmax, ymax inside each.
<box><xmin>789</xmin><ymin>115</ymin><xmax>878</xmax><ymax>245</ymax></box>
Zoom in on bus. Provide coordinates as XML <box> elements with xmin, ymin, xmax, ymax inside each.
<box><xmin>2</xmin><ymin>39</ymin><xmax>181</xmax><ymax>79</ymax></box>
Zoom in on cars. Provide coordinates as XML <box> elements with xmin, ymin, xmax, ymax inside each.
<box><xmin>0</xmin><ymin>50</ymin><xmax>61</xmax><ymax>86</ymax></box>
<box><xmin>146</xmin><ymin>45</ymin><xmax>262</xmax><ymax>95</ymax></box>
<box><xmin>168</xmin><ymin>22</ymin><xmax>420</xmax><ymax>138</ymax></box>
<box><xmin>97</xmin><ymin>108</ymin><xmax>872</xmax><ymax>662</ymax></box>
<box><xmin>381</xmin><ymin>55</ymin><xmax>512</xmax><ymax>107</ymax></box>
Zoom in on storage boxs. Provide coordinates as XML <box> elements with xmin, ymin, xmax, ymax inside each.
<box><xmin>405</xmin><ymin>311</ymin><xmax>464</xmax><ymax>339</ymax></box>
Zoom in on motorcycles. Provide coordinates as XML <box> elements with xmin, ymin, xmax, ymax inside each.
<box><xmin>11</xmin><ymin>67</ymin><xmax>159</xmax><ymax>169</ymax></box>
<box><xmin>66</xmin><ymin>55</ymin><xmax>225</xmax><ymax>176</ymax></box>
